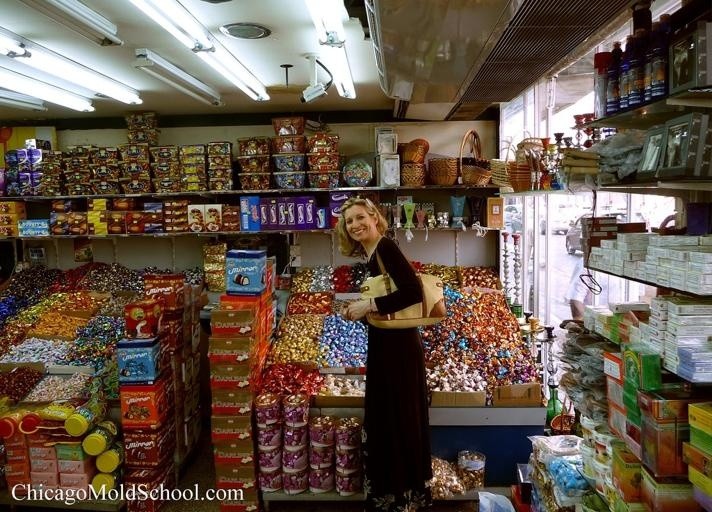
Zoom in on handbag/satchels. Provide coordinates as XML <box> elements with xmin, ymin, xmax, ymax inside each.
<box><xmin>360</xmin><ymin>245</ymin><xmax>448</xmax><ymax>330</ymax></box>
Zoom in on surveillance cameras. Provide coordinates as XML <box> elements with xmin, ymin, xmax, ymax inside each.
<box><xmin>298</xmin><ymin>82</ymin><xmax>329</xmax><ymax>104</ymax></box>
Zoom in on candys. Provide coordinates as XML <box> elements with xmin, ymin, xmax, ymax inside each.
<box><xmin>0</xmin><ymin>262</ymin><xmax>546</xmax><ymax>503</ymax></box>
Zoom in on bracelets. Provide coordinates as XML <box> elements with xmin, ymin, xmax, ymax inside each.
<box><xmin>369</xmin><ymin>297</ymin><xmax>373</xmax><ymax>312</ymax></box>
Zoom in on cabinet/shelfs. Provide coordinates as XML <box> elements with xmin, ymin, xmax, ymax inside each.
<box><xmin>0</xmin><ymin>135</ymin><xmax>544</xmax><ymax>241</ymax></box>
<box><xmin>542</xmin><ymin>1</ymin><xmax>711</xmax><ymax>510</ymax></box>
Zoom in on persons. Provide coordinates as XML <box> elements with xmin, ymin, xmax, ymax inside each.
<box><xmin>333</xmin><ymin>196</ymin><xmax>432</xmax><ymax>511</ymax></box>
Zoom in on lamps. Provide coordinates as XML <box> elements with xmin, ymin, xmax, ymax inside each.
<box><xmin>21</xmin><ymin>0</ymin><xmax>126</xmax><ymax>49</ymax></box>
<box><xmin>131</xmin><ymin>0</ymin><xmax>273</xmax><ymax>111</ymax></box>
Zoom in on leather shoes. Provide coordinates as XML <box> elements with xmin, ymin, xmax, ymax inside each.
<box><xmin>403</xmin><ymin>485</ymin><xmax>432</xmax><ymax>512</ymax></box>
<box><xmin>387</xmin><ymin>497</ymin><xmax>407</xmax><ymax>512</ymax></box>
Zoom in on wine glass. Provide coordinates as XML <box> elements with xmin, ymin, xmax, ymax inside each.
<box><xmin>378</xmin><ymin>195</ymin><xmax>487</xmax><ymax>230</ymax></box>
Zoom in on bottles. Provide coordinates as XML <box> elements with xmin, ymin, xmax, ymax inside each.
<box><xmin>3</xmin><ymin>398</ymin><xmax>124</xmax><ymax>496</ymax></box>
<box><xmin>459</xmin><ymin>450</ymin><xmax>485</xmax><ymax>492</ymax></box>
<box><xmin>546</xmin><ymin>390</ymin><xmax>566</xmax><ymax>425</ymax></box>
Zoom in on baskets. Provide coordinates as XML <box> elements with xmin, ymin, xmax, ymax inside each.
<box><xmin>551</xmin><ymin>393</ymin><xmax>576</xmax><ymax>435</ymax></box>
<box><xmin>459</xmin><ymin>130</ymin><xmax>492</xmax><ymax>186</ymax></box>
<box><xmin>429</xmin><ymin>154</ymin><xmax>458</xmax><ymax>186</ymax></box>
<box><xmin>398</xmin><ymin>139</ymin><xmax>430</xmax><ymax>187</ymax></box>
<box><xmin>491</xmin><ymin>135</ymin><xmax>543</xmax><ymax>193</ymax></box>
